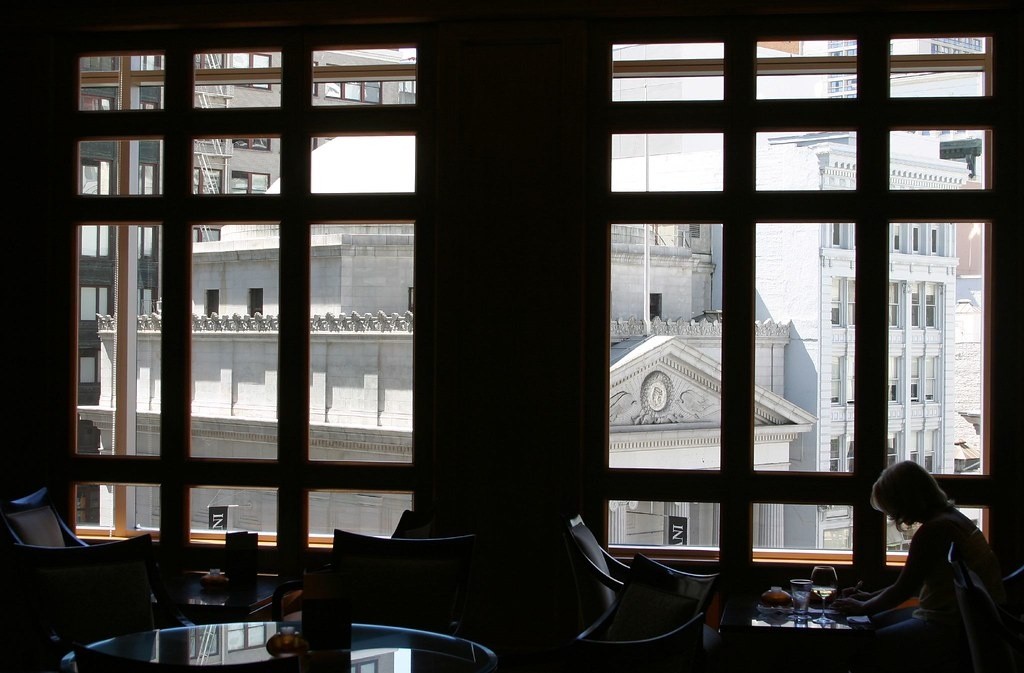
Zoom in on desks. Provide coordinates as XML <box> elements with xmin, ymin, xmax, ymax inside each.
<box><xmin>73</xmin><ymin>621</ymin><xmax>499</xmax><ymax>673</ymax></box>
<box><xmin>717</xmin><ymin>589</ymin><xmax>876</xmax><ymax>673</ymax></box>
<box><xmin>173</xmin><ymin>564</ymin><xmax>288</xmax><ymax>615</ymax></box>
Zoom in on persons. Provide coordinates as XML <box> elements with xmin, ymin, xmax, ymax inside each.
<box><xmin>830</xmin><ymin>462</ymin><xmax>1007</xmax><ymax>673</ymax></box>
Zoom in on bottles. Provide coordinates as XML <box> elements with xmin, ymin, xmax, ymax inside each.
<box><xmin>266</xmin><ymin>626</ymin><xmax>309</xmax><ymax>656</ymax></box>
<box><xmin>200</xmin><ymin>569</ymin><xmax>229</xmax><ymax>589</ymax></box>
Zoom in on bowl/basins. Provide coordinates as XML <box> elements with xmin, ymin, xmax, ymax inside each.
<box><xmin>757</xmin><ymin>586</ymin><xmax>792</xmax><ymax>608</ymax></box>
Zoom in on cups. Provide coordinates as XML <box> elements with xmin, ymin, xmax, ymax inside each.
<box><xmin>788</xmin><ymin>578</ymin><xmax>813</xmax><ymax>618</ymax></box>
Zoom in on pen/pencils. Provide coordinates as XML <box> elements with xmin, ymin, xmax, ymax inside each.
<box><xmin>845</xmin><ymin>579</ymin><xmax>864</xmax><ymax>598</ymax></box>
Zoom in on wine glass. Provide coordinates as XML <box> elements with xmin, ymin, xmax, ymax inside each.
<box><xmin>811</xmin><ymin>565</ymin><xmax>837</xmax><ymax>624</ymax></box>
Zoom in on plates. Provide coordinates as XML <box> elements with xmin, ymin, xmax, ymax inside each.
<box><xmin>757</xmin><ymin>608</ymin><xmax>794</xmax><ymax>613</ymax></box>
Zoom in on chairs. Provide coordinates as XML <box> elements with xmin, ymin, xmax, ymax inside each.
<box><xmin>947</xmin><ymin>542</ymin><xmax>1024</xmax><ymax>673</ymax></box>
<box><xmin>560</xmin><ymin>513</ymin><xmax>717</xmax><ymax>673</ymax></box>
<box><xmin>11</xmin><ymin>532</ymin><xmax>197</xmax><ymax>673</ymax></box>
<box><xmin>332</xmin><ymin>529</ymin><xmax>477</xmax><ymax>636</ymax></box>
<box><xmin>0</xmin><ymin>487</ymin><xmax>90</xmax><ymax>547</ymax></box>
